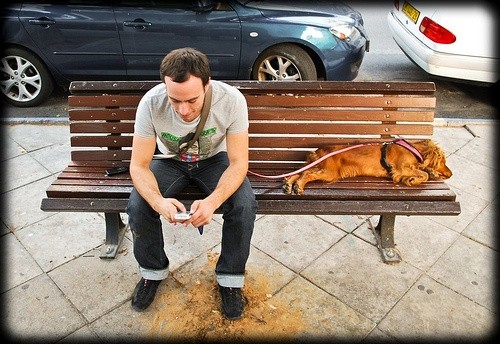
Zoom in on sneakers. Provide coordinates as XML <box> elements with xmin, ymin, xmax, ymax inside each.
<box><xmin>130</xmin><ymin>277</ymin><xmax>162</xmax><ymax>312</ymax></box>
<box><xmin>217</xmin><ymin>282</ymin><xmax>247</xmax><ymax>322</ymax></box>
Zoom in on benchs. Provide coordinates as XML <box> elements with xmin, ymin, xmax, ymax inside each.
<box><xmin>40</xmin><ymin>81</ymin><xmax>461</xmax><ymax>263</ymax></box>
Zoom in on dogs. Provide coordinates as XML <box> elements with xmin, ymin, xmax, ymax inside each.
<box><xmin>281</xmin><ymin>138</ymin><xmax>453</xmax><ymax>196</ymax></box>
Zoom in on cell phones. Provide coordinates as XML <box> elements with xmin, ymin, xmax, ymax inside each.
<box><xmin>105</xmin><ymin>166</ymin><xmax>128</xmax><ymax>176</ymax></box>
<box><xmin>174</xmin><ymin>209</ymin><xmax>192</xmax><ymax>221</ymax></box>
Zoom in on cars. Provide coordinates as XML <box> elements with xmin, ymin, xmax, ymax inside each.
<box><xmin>386</xmin><ymin>1</ymin><xmax>500</xmax><ymax>89</ymax></box>
<box><xmin>3</xmin><ymin>1</ymin><xmax>370</xmax><ymax>106</ymax></box>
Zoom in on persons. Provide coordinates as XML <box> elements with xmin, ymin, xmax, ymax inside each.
<box><xmin>125</xmin><ymin>46</ymin><xmax>257</xmax><ymax>320</ymax></box>
